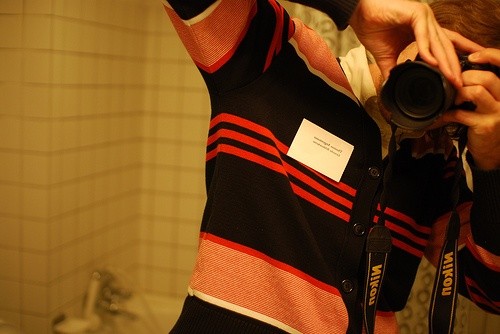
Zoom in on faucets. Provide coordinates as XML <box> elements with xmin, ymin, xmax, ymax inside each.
<box><xmin>81</xmin><ymin>269</ymin><xmax>139</xmax><ymax>330</ymax></box>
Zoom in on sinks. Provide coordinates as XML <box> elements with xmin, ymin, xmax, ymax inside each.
<box><xmin>61</xmin><ymin>287</ymin><xmax>190</xmax><ymax>333</ymax></box>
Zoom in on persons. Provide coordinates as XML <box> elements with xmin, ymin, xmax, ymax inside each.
<box><xmin>162</xmin><ymin>0</ymin><xmax>500</xmax><ymax>334</ymax></box>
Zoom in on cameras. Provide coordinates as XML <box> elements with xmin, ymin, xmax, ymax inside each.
<box><xmin>380</xmin><ymin>46</ymin><xmax>500</xmax><ymax>141</ymax></box>
